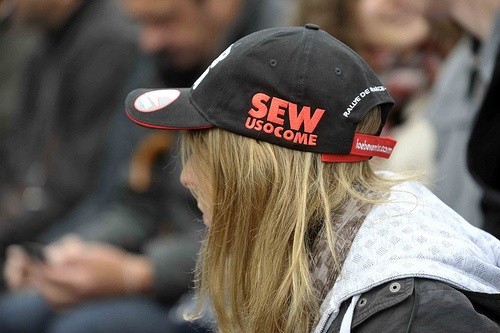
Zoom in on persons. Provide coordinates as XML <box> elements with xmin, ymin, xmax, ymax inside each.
<box><xmin>178</xmin><ymin>23</ymin><xmax>500</xmax><ymax>333</ymax></box>
<box><xmin>0</xmin><ymin>1</ymin><xmax>301</xmax><ymax>331</ymax></box>
<box><xmin>339</xmin><ymin>0</ymin><xmax>453</xmax><ymax>125</ymax></box>
<box><xmin>384</xmin><ymin>0</ymin><xmax>500</xmax><ymax>241</ymax></box>
<box><xmin>2</xmin><ymin>0</ymin><xmax>158</xmax><ymax>240</ymax></box>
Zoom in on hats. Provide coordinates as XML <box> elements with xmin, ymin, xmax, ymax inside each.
<box><xmin>122</xmin><ymin>22</ymin><xmax>395</xmax><ymax>160</ymax></box>
